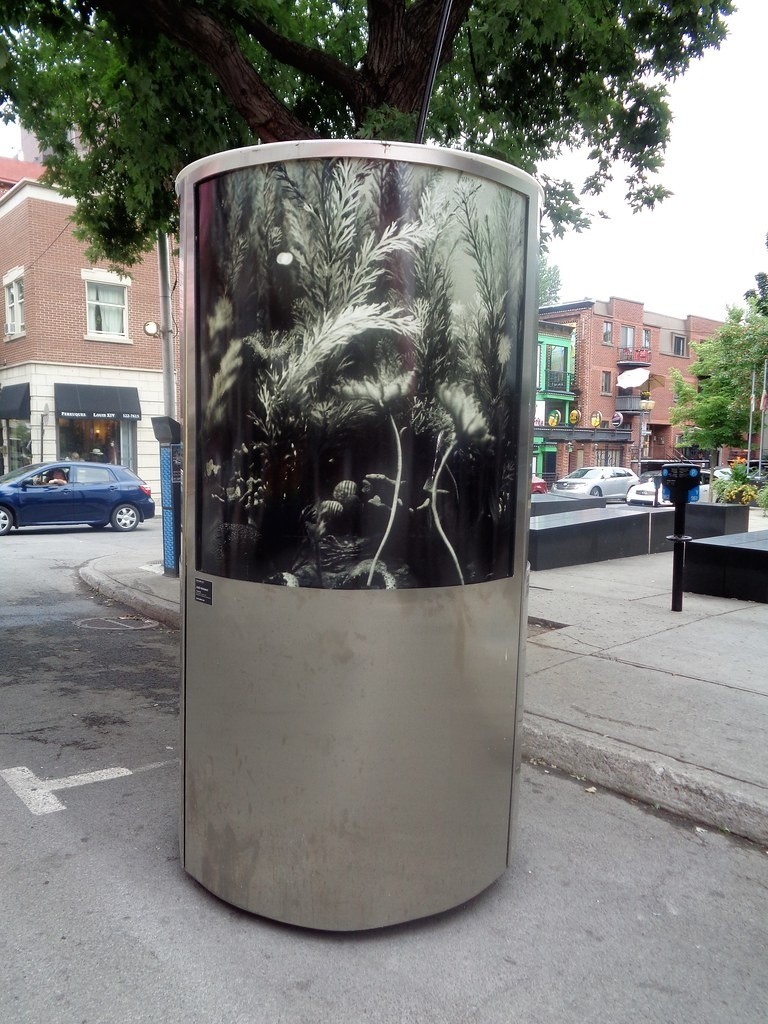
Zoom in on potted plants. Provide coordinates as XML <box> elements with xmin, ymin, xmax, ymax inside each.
<box><xmin>756</xmin><ymin>485</ymin><xmax>768</xmax><ymax>517</ymax></box>
<box><xmin>711</xmin><ymin>456</ymin><xmax>757</xmax><ymax>506</ymax></box>
<box><xmin>640</xmin><ymin>390</ymin><xmax>651</xmax><ymax>400</ymax></box>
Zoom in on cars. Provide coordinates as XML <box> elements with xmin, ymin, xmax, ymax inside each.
<box><xmin>0</xmin><ymin>460</ymin><xmax>155</xmax><ymax>535</ymax></box>
<box><xmin>626</xmin><ymin>466</ymin><xmax>768</xmax><ymax>506</ymax></box>
<box><xmin>531</xmin><ymin>476</ymin><xmax>547</xmax><ymax>494</ymax></box>
<box><xmin>551</xmin><ymin>466</ymin><xmax>641</xmax><ymax>504</ymax></box>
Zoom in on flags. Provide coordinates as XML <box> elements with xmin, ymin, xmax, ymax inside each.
<box><xmin>751</xmin><ymin>395</ymin><xmax>755</xmax><ymax>413</ymax></box>
<box><xmin>760</xmin><ymin>390</ymin><xmax>767</xmax><ymax>413</ymax></box>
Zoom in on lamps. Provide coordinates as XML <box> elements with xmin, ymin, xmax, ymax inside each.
<box><xmin>631</xmin><ymin>444</ymin><xmax>634</xmax><ymax>450</ymax></box>
<box><xmin>594</xmin><ymin>443</ymin><xmax>598</xmax><ymax>450</ymax></box>
<box><xmin>96</xmin><ymin>428</ymin><xmax>100</xmax><ymax>437</ymax></box>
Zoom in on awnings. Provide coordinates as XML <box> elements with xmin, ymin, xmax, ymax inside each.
<box><xmin>54</xmin><ymin>383</ymin><xmax>141</xmax><ymax>421</ymax></box>
<box><xmin>0</xmin><ymin>383</ymin><xmax>30</xmax><ymax>419</ymax></box>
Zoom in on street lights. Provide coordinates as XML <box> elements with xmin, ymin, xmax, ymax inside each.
<box><xmin>638</xmin><ymin>400</ymin><xmax>655</xmax><ymax>479</ymax></box>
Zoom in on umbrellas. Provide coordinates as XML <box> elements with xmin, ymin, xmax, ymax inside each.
<box><xmin>616</xmin><ymin>367</ymin><xmax>666</xmax><ymax>391</ymax></box>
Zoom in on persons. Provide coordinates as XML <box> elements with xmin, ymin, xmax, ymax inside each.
<box><xmin>95</xmin><ymin>434</ymin><xmax>104</xmax><ymax>452</ymax></box>
<box><xmin>71</xmin><ymin>451</ymin><xmax>79</xmax><ymax>461</ymax></box>
<box><xmin>49</xmin><ymin>469</ymin><xmax>67</xmax><ymax>485</ymax></box>
<box><xmin>85</xmin><ymin>449</ymin><xmax>104</xmax><ymax>462</ymax></box>
